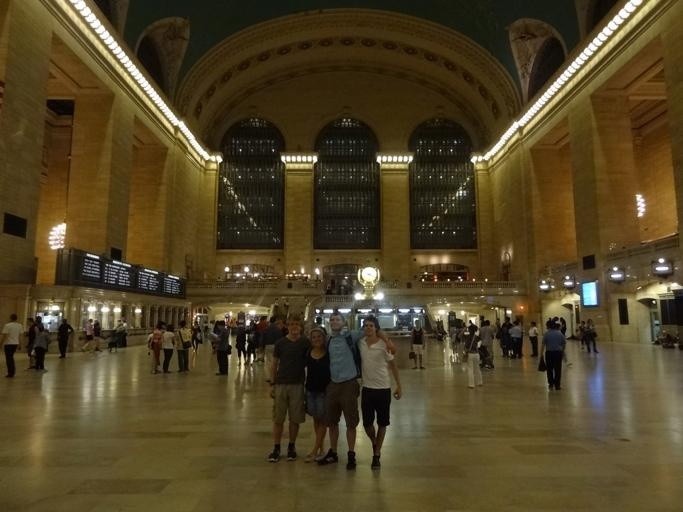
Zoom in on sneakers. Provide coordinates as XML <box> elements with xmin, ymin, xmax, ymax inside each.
<box><xmin>269</xmin><ymin>444</ymin><xmax>381</xmax><ymax>468</ymax></box>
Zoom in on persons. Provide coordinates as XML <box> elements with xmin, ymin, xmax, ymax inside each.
<box><xmin>354</xmin><ymin>315</ymin><xmax>401</xmax><ymax>471</ymax></box>
<box><xmin>208</xmin><ymin>315</ymin><xmax>268</xmax><ymax>374</ymax></box>
<box><xmin>82</xmin><ymin>319</ymin><xmax>127</xmax><ymax>352</ymax></box>
<box><xmin>651</xmin><ymin>329</ymin><xmax>682</xmax><ymax>350</ymax></box>
<box><xmin>312</xmin><ymin>317</ymin><xmax>327</xmax><ymax>335</ymax></box>
<box><xmin>265</xmin><ymin>314</ymin><xmax>311</xmax><ymax>464</ymax></box>
<box><xmin>579</xmin><ymin>319</ymin><xmax>598</xmax><ymax>353</ymax></box>
<box><xmin>147</xmin><ymin>321</ymin><xmax>201</xmax><ymax>374</ymax></box>
<box><xmin>304</xmin><ymin>326</ymin><xmax>330</xmax><ymax>464</ymax></box>
<box><xmin>410</xmin><ymin>320</ymin><xmax>426</xmax><ymax>369</ymax></box>
<box><xmin>437</xmin><ymin>320</ymin><xmax>445</xmax><ymax>340</ymax></box>
<box><xmin>496</xmin><ymin>316</ymin><xmax>523</xmax><ymax>358</ymax></box>
<box><xmin>529</xmin><ymin>321</ymin><xmax>539</xmax><ymax>358</ymax></box>
<box><xmin>452</xmin><ymin>315</ymin><xmax>494</xmax><ymax>368</ymax></box>
<box><xmin>26</xmin><ymin>316</ymin><xmax>51</xmax><ymax>369</ymax></box>
<box><xmin>540</xmin><ymin>316</ymin><xmax>567</xmax><ymax>391</ymax></box>
<box><xmin>3</xmin><ymin>314</ymin><xmax>23</xmax><ymax>377</ymax></box>
<box><xmin>58</xmin><ymin>319</ymin><xmax>73</xmax><ymax>358</ymax></box>
<box><xmin>316</xmin><ymin>311</ymin><xmax>397</xmax><ymax>470</ymax></box>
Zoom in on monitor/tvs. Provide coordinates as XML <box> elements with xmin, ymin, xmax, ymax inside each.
<box><xmin>581</xmin><ymin>282</ymin><xmax>599</xmax><ymax>307</ymax></box>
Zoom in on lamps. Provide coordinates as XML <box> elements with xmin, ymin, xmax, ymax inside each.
<box><xmin>46</xmin><ymin>100</ymin><xmax>76</xmax><ymax>252</ymax></box>
<box><xmin>533</xmin><ymin>255</ymin><xmax>676</xmax><ymax>294</ymax></box>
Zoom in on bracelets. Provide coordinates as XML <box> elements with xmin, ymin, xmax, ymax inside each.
<box><xmin>269</xmin><ymin>382</ymin><xmax>275</xmax><ymax>386</ymax></box>
